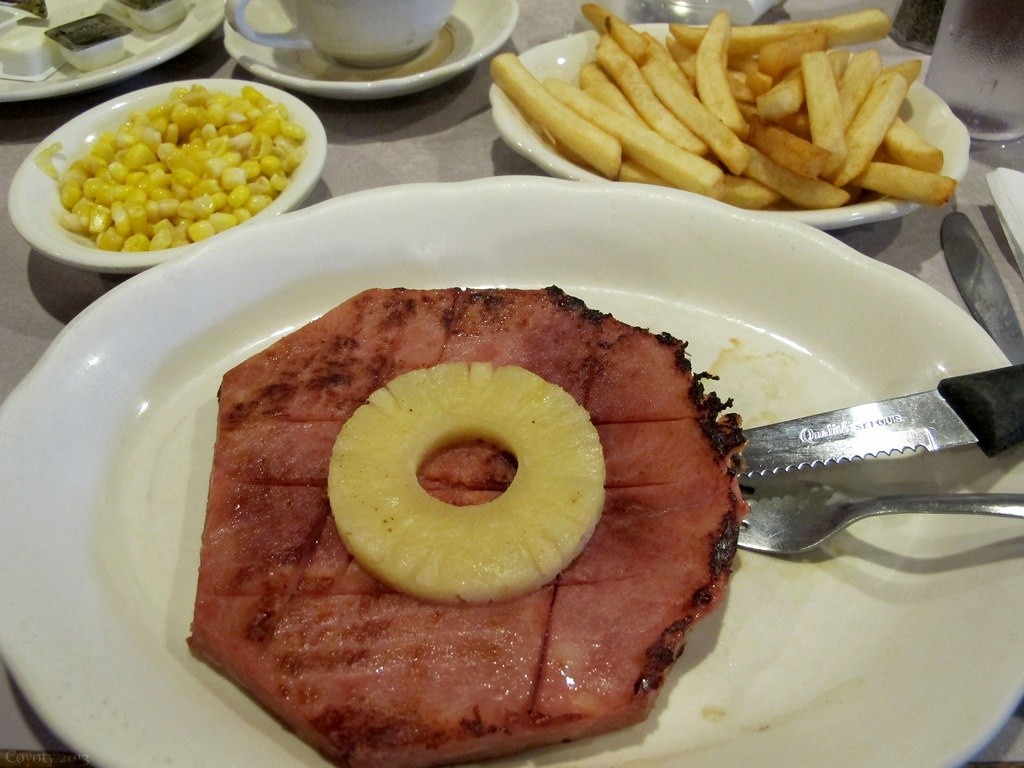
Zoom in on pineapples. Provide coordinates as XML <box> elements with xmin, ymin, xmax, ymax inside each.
<box><xmin>326</xmin><ymin>360</ymin><xmax>606</xmax><ymax>603</ymax></box>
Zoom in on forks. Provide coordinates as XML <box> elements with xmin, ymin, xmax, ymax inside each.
<box><xmin>734</xmin><ymin>476</ymin><xmax>1024</xmax><ymax>555</ymax></box>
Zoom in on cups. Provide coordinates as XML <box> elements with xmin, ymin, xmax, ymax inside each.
<box><xmin>924</xmin><ymin>0</ymin><xmax>1024</xmax><ymax>147</ymax></box>
<box><xmin>225</xmin><ymin>0</ymin><xmax>457</xmax><ymax>69</ymax></box>
<box><xmin>638</xmin><ymin>1</ymin><xmax>783</xmax><ymax>30</ymax></box>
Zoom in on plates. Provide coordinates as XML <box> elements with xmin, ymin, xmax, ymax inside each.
<box><xmin>9</xmin><ymin>78</ymin><xmax>327</xmax><ymax>273</ymax></box>
<box><xmin>0</xmin><ymin>0</ymin><xmax>224</xmax><ymax>102</ymax></box>
<box><xmin>222</xmin><ymin>0</ymin><xmax>517</xmax><ymax>100</ymax></box>
<box><xmin>490</xmin><ymin>22</ymin><xmax>971</xmax><ymax>230</ymax></box>
<box><xmin>0</xmin><ymin>175</ymin><xmax>1024</xmax><ymax>768</ymax></box>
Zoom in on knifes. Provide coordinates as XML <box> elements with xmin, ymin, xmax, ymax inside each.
<box><xmin>728</xmin><ymin>362</ymin><xmax>1024</xmax><ymax>478</ymax></box>
<box><xmin>941</xmin><ymin>212</ymin><xmax>1024</xmax><ymax>365</ymax></box>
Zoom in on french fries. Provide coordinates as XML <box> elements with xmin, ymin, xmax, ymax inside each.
<box><xmin>488</xmin><ymin>3</ymin><xmax>956</xmax><ymax>211</ymax></box>
